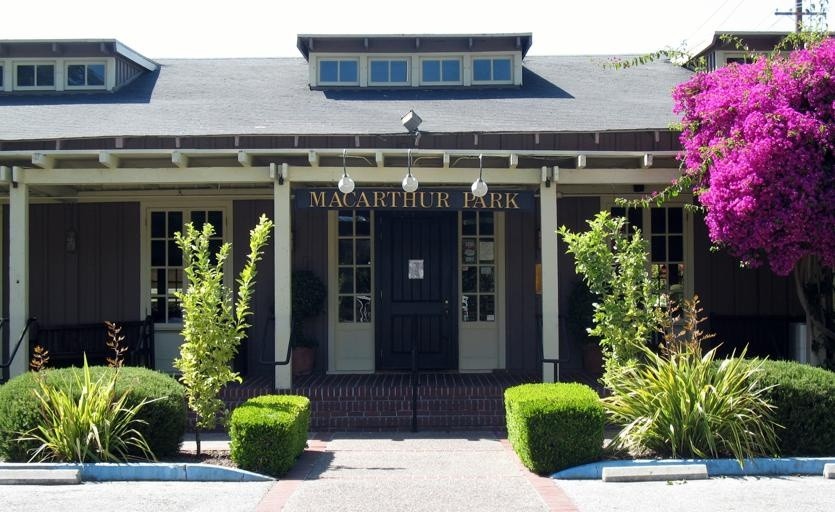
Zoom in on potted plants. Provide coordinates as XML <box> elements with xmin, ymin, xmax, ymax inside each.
<box><xmin>566</xmin><ymin>275</ymin><xmax>612</xmax><ymax>375</ymax></box>
<box><xmin>269</xmin><ymin>271</ymin><xmax>328</xmax><ymax>376</ymax></box>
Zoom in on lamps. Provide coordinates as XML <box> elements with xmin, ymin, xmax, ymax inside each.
<box><xmin>338</xmin><ymin>149</ymin><xmax>355</xmax><ymax>194</ymax></box>
<box><xmin>471</xmin><ymin>153</ymin><xmax>489</xmax><ymax>197</ymax></box>
<box><xmin>401</xmin><ymin>110</ymin><xmax>422</xmax><ymax>133</ymax></box>
<box><xmin>402</xmin><ymin>149</ymin><xmax>418</xmax><ymax>193</ymax></box>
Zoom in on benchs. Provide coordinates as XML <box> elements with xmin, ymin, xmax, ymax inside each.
<box><xmin>32</xmin><ymin>315</ymin><xmax>155</xmax><ymax>370</ymax></box>
